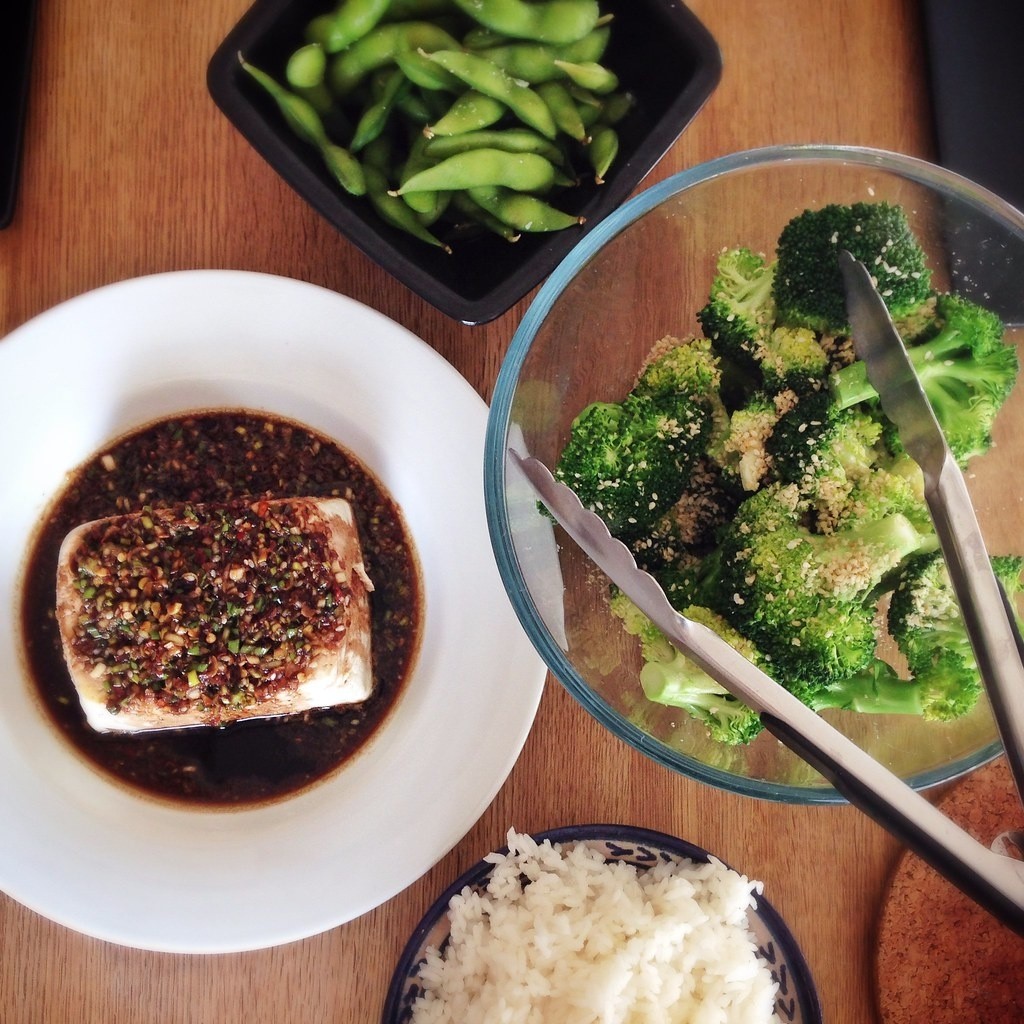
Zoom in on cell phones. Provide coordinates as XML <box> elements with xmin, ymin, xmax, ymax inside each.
<box><xmin>924</xmin><ymin>0</ymin><xmax>1024</xmax><ymax>328</ymax></box>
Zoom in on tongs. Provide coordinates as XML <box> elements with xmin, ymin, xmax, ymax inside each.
<box><xmin>508</xmin><ymin>250</ymin><xmax>1024</xmax><ymax>912</ymax></box>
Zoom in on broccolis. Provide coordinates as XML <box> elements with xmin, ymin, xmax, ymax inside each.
<box><xmin>536</xmin><ymin>198</ymin><xmax>1024</xmax><ymax>751</ymax></box>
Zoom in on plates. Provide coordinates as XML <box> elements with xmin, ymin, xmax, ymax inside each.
<box><xmin>921</xmin><ymin>0</ymin><xmax>1024</xmax><ymax>215</ymax></box>
<box><xmin>0</xmin><ymin>0</ymin><xmax>39</xmax><ymax>231</ymax></box>
<box><xmin>0</xmin><ymin>270</ymin><xmax>548</xmax><ymax>954</ymax></box>
<box><xmin>484</xmin><ymin>143</ymin><xmax>1024</xmax><ymax>807</ymax></box>
<box><xmin>207</xmin><ymin>0</ymin><xmax>722</xmax><ymax>326</ymax></box>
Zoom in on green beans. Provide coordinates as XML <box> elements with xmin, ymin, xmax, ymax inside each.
<box><xmin>236</xmin><ymin>0</ymin><xmax>636</xmax><ymax>249</ymax></box>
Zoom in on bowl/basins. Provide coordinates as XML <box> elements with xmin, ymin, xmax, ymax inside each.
<box><xmin>381</xmin><ymin>824</ymin><xmax>822</xmax><ymax>1024</ymax></box>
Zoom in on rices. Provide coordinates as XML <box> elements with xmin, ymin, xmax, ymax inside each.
<box><xmin>408</xmin><ymin>825</ymin><xmax>785</xmax><ymax>1024</ymax></box>
